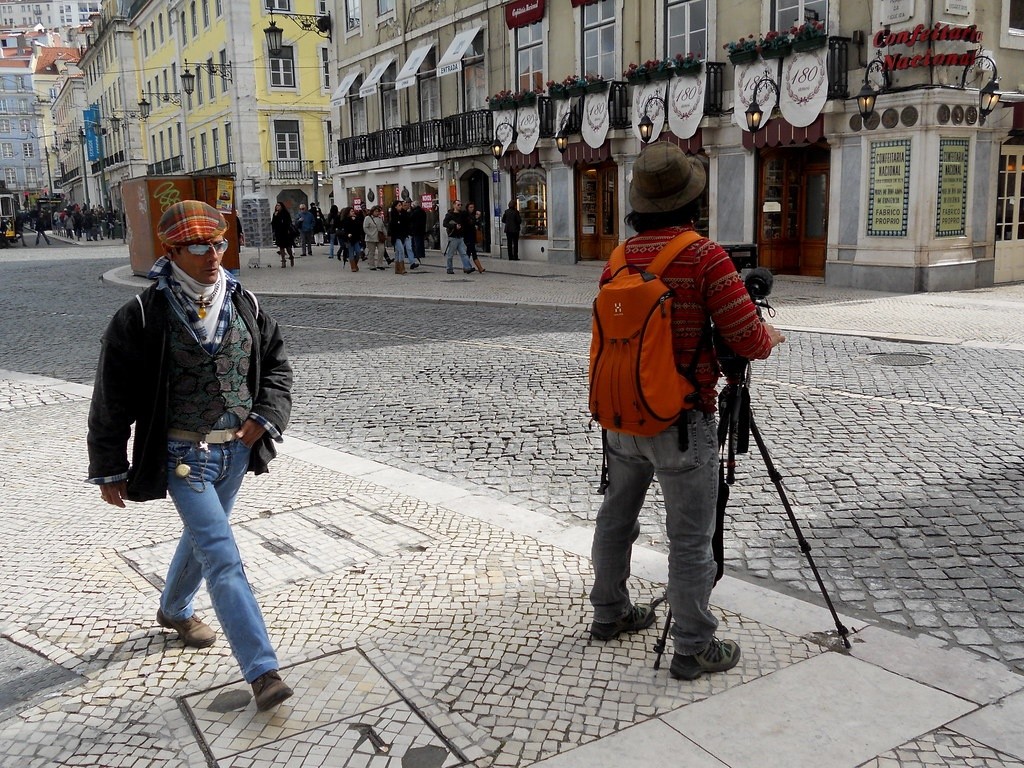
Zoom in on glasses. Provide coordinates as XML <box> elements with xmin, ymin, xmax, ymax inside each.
<box><xmin>180</xmin><ymin>240</ymin><xmax>229</xmax><ymax>255</ymax></box>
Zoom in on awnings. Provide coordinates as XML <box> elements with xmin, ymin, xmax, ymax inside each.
<box><xmin>435</xmin><ymin>23</ymin><xmax>482</xmax><ymax>78</ymax></box>
<box><xmin>390</xmin><ymin>43</ymin><xmax>435</xmax><ymax>89</ymax></box>
<box><xmin>356</xmin><ymin>57</ymin><xmax>397</xmax><ymax>99</ymax></box>
<box><xmin>325</xmin><ymin>70</ymin><xmax>362</xmax><ymax>108</ymax></box>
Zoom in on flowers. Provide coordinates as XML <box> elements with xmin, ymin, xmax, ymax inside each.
<box><xmin>622</xmin><ymin>51</ymin><xmax>703</xmax><ymax>77</ymax></box>
<box><xmin>543</xmin><ymin>72</ymin><xmax>605</xmax><ymax>90</ymax></box>
<box><xmin>722</xmin><ymin>18</ymin><xmax>826</xmax><ymax>54</ymax></box>
<box><xmin>485</xmin><ymin>88</ymin><xmax>541</xmax><ymax>102</ymax></box>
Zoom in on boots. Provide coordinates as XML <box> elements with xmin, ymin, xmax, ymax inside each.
<box><xmin>350</xmin><ymin>260</ymin><xmax>359</xmax><ymax>272</ymax></box>
<box><xmin>399</xmin><ymin>261</ymin><xmax>407</xmax><ymax>274</ymax></box>
<box><xmin>290</xmin><ymin>257</ymin><xmax>294</xmax><ymax>266</ymax></box>
<box><xmin>385</xmin><ymin>256</ymin><xmax>395</xmax><ymax>265</ymax></box>
<box><xmin>280</xmin><ymin>260</ymin><xmax>286</xmax><ymax>267</ymax></box>
<box><xmin>394</xmin><ymin>261</ymin><xmax>401</xmax><ymax>273</ymax></box>
<box><xmin>474</xmin><ymin>259</ymin><xmax>485</xmax><ymax>273</ymax></box>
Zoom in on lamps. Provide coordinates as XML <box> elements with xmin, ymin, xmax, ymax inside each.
<box><xmin>488</xmin><ymin>123</ymin><xmax>518</xmax><ymax>161</ymax></box>
<box><xmin>959</xmin><ymin>49</ymin><xmax>1003</xmax><ymax>119</ymax></box>
<box><xmin>637</xmin><ymin>97</ymin><xmax>669</xmax><ymax>145</ymax></box>
<box><xmin>263</xmin><ymin>6</ymin><xmax>333</xmax><ymax>58</ymax></box>
<box><xmin>744</xmin><ymin>67</ymin><xmax>780</xmax><ymax>135</ymax></box>
<box><xmin>854</xmin><ymin>48</ymin><xmax>892</xmax><ymax>121</ymax></box>
<box><xmin>49</xmin><ymin>122</ymin><xmax>102</xmax><ymax>153</ymax></box>
<box><xmin>137</xmin><ymin>90</ymin><xmax>183</xmax><ymax>122</ymax></box>
<box><xmin>178</xmin><ymin>57</ymin><xmax>234</xmax><ymax>96</ymax></box>
<box><xmin>107</xmin><ymin>107</ymin><xmax>143</xmax><ymax>132</ymax></box>
<box><xmin>555</xmin><ymin>111</ymin><xmax>585</xmax><ymax>155</ymax></box>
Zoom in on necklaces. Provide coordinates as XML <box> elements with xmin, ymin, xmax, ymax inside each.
<box><xmin>185</xmin><ymin>279</ymin><xmax>222</xmax><ymax>319</ymax></box>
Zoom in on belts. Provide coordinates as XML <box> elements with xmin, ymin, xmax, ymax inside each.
<box><xmin>169</xmin><ymin>425</ymin><xmax>240</xmax><ymax>443</ymax></box>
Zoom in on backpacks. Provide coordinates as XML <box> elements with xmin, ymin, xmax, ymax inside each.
<box><xmin>589</xmin><ymin>231</ymin><xmax>715</xmax><ymax>435</ymax></box>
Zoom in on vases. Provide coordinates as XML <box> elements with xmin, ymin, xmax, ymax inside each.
<box><xmin>550</xmin><ymin>83</ymin><xmax>607</xmax><ymax>99</ymax></box>
<box><xmin>627</xmin><ymin>64</ymin><xmax>701</xmax><ymax>87</ymax></box>
<box><xmin>727</xmin><ymin>35</ymin><xmax>827</xmax><ymax>66</ymax></box>
<box><xmin>489</xmin><ymin>101</ymin><xmax>535</xmax><ymax>111</ymax></box>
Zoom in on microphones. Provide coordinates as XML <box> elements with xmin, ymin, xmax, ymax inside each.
<box><xmin>744</xmin><ymin>267</ymin><xmax>774</xmax><ymax>297</ymax></box>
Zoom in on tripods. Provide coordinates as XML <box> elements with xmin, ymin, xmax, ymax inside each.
<box><xmin>652</xmin><ymin>270</ymin><xmax>851</xmax><ymax>671</ymax></box>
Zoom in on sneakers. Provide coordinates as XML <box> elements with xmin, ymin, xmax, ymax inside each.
<box><xmin>156</xmin><ymin>608</ymin><xmax>214</xmax><ymax>648</ymax></box>
<box><xmin>410</xmin><ymin>263</ymin><xmax>420</xmax><ymax>270</ymax></box>
<box><xmin>669</xmin><ymin>637</ymin><xmax>742</xmax><ymax>680</ymax></box>
<box><xmin>591</xmin><ymin>606</ymin><xmax>657</xmax><ymax>637</ymax></box>
<box><xmin>250</xmin><ymin>669</ymin><xmax>294</xmax><ymax>713</ymax></box>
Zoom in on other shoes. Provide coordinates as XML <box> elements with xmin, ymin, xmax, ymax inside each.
<box><xmin>509</xmin><ymin>257</ymin><xmax>521</xmax><ymax>261</ymax></box>
<box><xmin>300</xmin><ymin>254</ymin><xmax>306</xmax><ymax>256</ymax></box>
<box><xmin>377</xmin><ymin>267</ymin><xmax>385</xmax><ymax>270</ymax></box>
<box><xmin>308</xmin><ymin>253</ymin><xmax>312</xmax><ymax>256</ymax></box>
<box><xmin>371</xmin><ymin>268</ymin><xmax>378</xmax><ymax>270</ymax></box>
<box><xmin>2</xmin><ymin>230</ymin><xmax>122</xmax><ymax>246</ymax></box>
<box><xmin>464</xmin><ymin>267</ymin><xmax>476</xmax><ymax>274</ymax></box>
<box><xmin>335</xmin><ymin>250</ymin><xmax>368</xmax><ymax>264</ymax></box>
<box><xmin>328</xmin><ymin>256</ymin><xmax>333</xmax><ymax>258</ymax></box>
<box><xmin>447</xmin><ymin>269</ymin><xmax>454</xmax><ymax>274</ymax></box>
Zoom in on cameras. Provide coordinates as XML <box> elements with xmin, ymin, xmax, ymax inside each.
<box><xmin>710</xmin><ymin>241</ymin><xmax>759</xmax><ymax>270</ymax></box>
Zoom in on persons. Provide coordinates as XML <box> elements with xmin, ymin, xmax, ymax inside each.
<box><xmin>271</xmin><ymin>202</ymin><xmax>296</xmax><ymax>269</ymax></box>
<box><xmin>2</xmin><ymin>201</ymin><xmax>118</xmax><ymax>249</ymax></box>
<box><xmin>501</xmin><ymin>200</ymin><xmax>523</xmax><ymax>261</ymax></box>
<box><xmin>86</xmin><ymin>198</ymin><xmax>296</xmax><ymax>713</ymax></box>
<box><xmin>294</xmin><ymin>196</ymin><xmax>488</xmax><ymax>276</ymax></box>
<box><xmin>234</xmin><ymin>209</ymin><xmax>245</xmax><ymax>253</ymax></box>
<box><xmin>584</xmin><ymin>139</ymin><xmax>790</xmax><ymax>673</ymax></box>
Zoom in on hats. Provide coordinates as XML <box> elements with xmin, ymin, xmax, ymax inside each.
<box><xmin>630</xmin><ymin>140</ymin><xmax>706</xmax><ymax>211</ymax></box>
<box><xmin>157</xmin><ymin>198</ymin><xmax>229</xmax><ymax>244</ymax></box>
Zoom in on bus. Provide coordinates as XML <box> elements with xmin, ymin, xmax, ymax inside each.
<box><xmin>0</xmin><ymin>180</ymin><xmax>19</xmax><ymax>243</ymax></box>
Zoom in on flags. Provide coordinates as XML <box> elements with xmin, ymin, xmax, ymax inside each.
<box><xmin>80</xmin><ymin>105</ymin><xmax>104</xmax><ymax>161</ymax></box>
